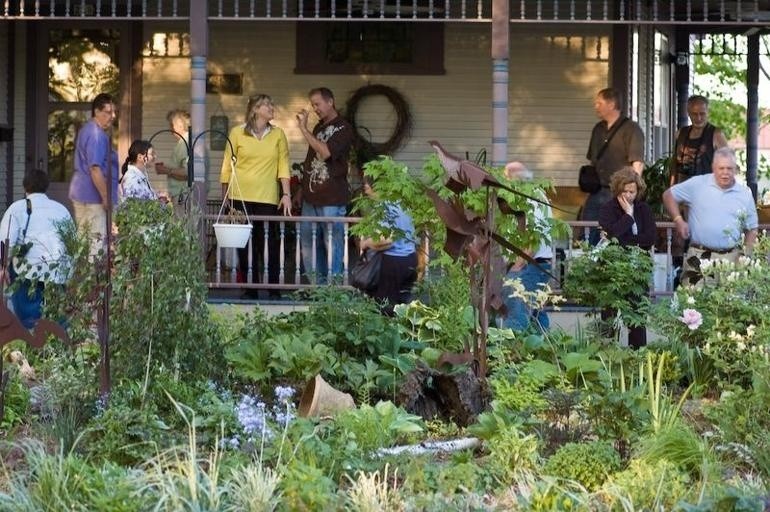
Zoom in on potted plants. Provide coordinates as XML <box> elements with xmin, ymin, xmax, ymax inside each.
<box><xmin>212</xmin><ymin>208</ymin><xmax>253</xmax><ymax>250</ymax></box>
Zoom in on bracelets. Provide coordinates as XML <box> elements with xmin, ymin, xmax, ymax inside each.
<box><xmin>283</xmin><ymin>192</ymin><xmax>291</xmax><ymax>197</ymax></box>
<box><xmin>166</xmin><ymin>168</ymin><xmax>173</xmax><ymax>177</ymax></box>
<box><xmin>674</xmin><ymin>215</ymin><xmax>683</xmax><ymax>222</ymax></box>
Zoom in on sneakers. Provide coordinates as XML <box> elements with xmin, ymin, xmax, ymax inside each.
<box><xmin>240</xmin><ymin>287</ymin><xmax>283</xmax><ymax>300</ymax></box>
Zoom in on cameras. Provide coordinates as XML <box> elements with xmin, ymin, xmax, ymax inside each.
<box><xmin>674</xmin><ymin>162</ymin><xmax>690</xmax><ymax>174</ymax></box>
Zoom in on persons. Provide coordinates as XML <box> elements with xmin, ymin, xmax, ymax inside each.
<box><xmin>291</xmin><ymin>87</ymin><xmax>355</xmax><ymax>285</ymax></box>
<box><xmin>582</xmin><ymin>88</ymin><xmax>645</xmax><ymax>245</ymax></box>
<box><xmin>501</xmin><ymin>160</ymin><xmax>554</xmax><ymax>334</ymax></box>
<box><xmin>219</xmin><ymin>94</ymin><xmax>292</xmax><ymax>300</ymax></box>
<box><xmin>155</xmin><ymin>109</ymin><xmax>209</xmax><ymax>258</ymax></box>
<box><xmin>120</xmin><ymin>140</ymin><xmax>158</xmax><ymax>200</ymax></box>
<box><xmin>598</xmin><ymin>167</ymin><xmax>657</xmax><ymax>350</ymax></box>
<box><xmin>0</xmin><ymin>169</ymin><xmax>76</xmax><ymax>334</ymax></box>
<box><xmin>668</xmin><ymin>95</ymin><xmax>728</xmax><ymax>293</ymax></box>
<box><xmin>68</xmin><ymin>93</ymin><xmax>118</xmax><ymax>265</ymax></box>
<box><xmin>662</xmin><ymin>147</ymin><xmax>759</xmax><ymax>285</ymax></box>
<box><xmin>359</xmin><ymin>176</ymin><xmax>418</xmax><ymax>317</ymax></box>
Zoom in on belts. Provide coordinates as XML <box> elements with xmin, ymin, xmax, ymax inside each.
<box><xmin>535</xmin><ymin>258</ymin><xmax>553</xmax><ymax>264</ymax></box>
<box><xmin>689</xmin><ymin>242</ymin><xmax>738</xmax><ymax>254</ymax></box>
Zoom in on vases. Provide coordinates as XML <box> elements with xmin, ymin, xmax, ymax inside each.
<box><xmin>296</xmin><ymin>373</ymin><xmax>357</xmax><ymax>422</ymax></box>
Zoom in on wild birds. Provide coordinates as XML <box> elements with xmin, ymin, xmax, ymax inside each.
<box><xmin>424</xmin><ymin>188</ymin><xmax>561</xmax><ymax>376</ymax></box>
<box><xmin>427</xmin><ymin>137</ymin><xmax>580</xmax><ymax>377</ymax></box>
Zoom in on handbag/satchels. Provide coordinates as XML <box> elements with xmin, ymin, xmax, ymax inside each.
<box><xmin>349</xmin><ymin>254</ymin><xmax>383</xmax><ymax>290</ymax></box>
<box><xmin>578</xmin><ymin>164</ymin><xmax>603</xmax><ymax>194</ymax></box>
<box><xmin>3</xmin><ymin>264</ymin><xmax>45</xmax><ymax>332</ymax></box>
